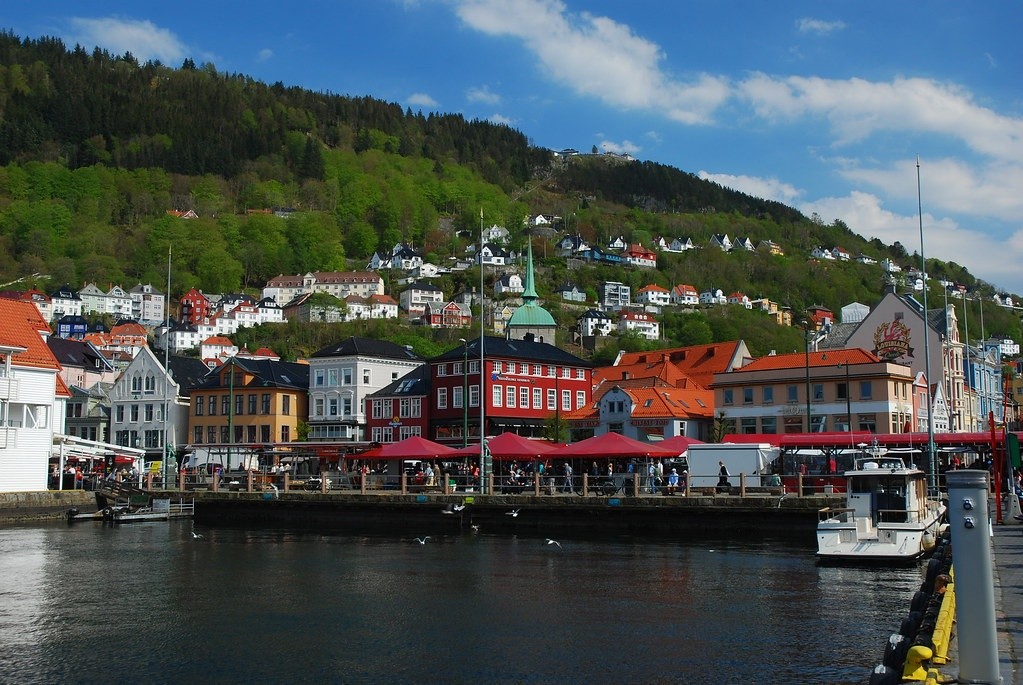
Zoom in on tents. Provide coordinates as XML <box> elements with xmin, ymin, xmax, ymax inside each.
<box><xmin>356</xmin><ymin>432</ymin><xmax>706</xmax><ymax>477</ymax></box>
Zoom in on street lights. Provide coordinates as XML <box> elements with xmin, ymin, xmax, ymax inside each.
<box><xmin>458</xmin><ymin>338</ymin><xmax>469</xmax><ymax>449</ymax></box>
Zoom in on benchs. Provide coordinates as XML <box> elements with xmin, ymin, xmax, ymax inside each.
<box><xmin>690</xmin><ymin>487</ymin><xmax>717</xmax><ymax>496</ymax></box>
<box><xmin>745</xmin><ymin>486</ymin><xmax>782</xmax><ymax>496</ymax></box>
<box><xmin>517</xmin><ymin>485</ymin><xmax>550</xmax><ymax>495</ymax></box>
<box><xmin>409</xmin><ymin>486</ymin><xmax>453</xmax><ymax>494</ymax></box>
<box><xmin>184</xmin><ymin>483</ymin><xmax>213</xmax><ymax>491</ymax></box>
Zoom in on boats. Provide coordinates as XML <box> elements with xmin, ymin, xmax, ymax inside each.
<box><xmin>813</xmin><ymin>415</ymin><xmax>948</xmax><ymax>565</ymax></box>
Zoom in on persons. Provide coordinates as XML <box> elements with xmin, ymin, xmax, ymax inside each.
<box><xmin>770</xmin><ymin>473</ymin><xmax>786</xmax><ymax>494</ymax></box>
<box><xmin>239</xmin><ymin>462</ymin><xmax>245</xmax><ymax>471</ymax></box>
<box><xmin>175</xmin><ymin>466</ymin><xmax>208</xmax><ymax>483</ymax></box>
<box><xmin>57</xmin><ymin>463</ymin><xmax>136</xmax><ymax>486</ymax></box>
<box><xmin>271</xmin><ymin>462</ymin><xmax>291</xmax><ymax>475</ymax></box>
<box><xmin>362</xmin><ymin>460</ymin><xmax>687</xmax><ymax>495</ymax></box>
<box><xmin>717</xmin><ymin>461</ymin><xmax>731</xmax><ymax>494</ymax></box>
<box><xmin>218</xmin><ymin>465</ymin><xmax>226</xmax><ymax>488</ymax></box>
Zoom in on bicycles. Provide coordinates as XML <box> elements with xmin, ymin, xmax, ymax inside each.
<box><xmin>303</xmin><ymin>480</ymin><xmax>332</xmax><ymax>493</ymax></box>
<box><xmin>575</xmin><ymin>476</ymin><xmax>634</xmax><ymax>497</ymax></box>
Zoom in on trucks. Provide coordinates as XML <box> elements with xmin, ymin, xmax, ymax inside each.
<box><xmin>181</xmin><ymin>449</ymin><xmax>259</xmax><ymax>472</ymax></box>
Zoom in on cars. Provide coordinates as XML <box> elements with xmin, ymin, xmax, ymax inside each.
<box><xmin>183</xmin><ymin>462</ymin><xmax>224</xmax><ymax>474</ymax></box>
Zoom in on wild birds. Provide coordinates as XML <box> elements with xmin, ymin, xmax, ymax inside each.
<box><xmin>413</xmin><ymin>536</ymin><xmax>431</xmax><ymax>545</ymax></box>
<box><xmin>454</xmin><ymin>504</ymin><xmax>466</xmax><ymax>511</ymax></box>
<box><xmin>542</xmin><ymin>538</ymin><xmax>562</xmax><ymax>550</ymax></box>
<box><xmin>505</xmin><ymin>509</ymin><xmax>520</xmax><ymax>518</ymax></box>
<box><xmin>191</xmin><ymin>532</ymin><xmax>203</xmax><ymax>538</ymax></box>
<box><xmin>270</xmin><ymin>483</ymin><xmax>278</xmax><ymax>498</ymax></box>
<box><xmin>441</xmin><ymin>503</ymin><xmax>454</xmax><ymax>514</ymax></box>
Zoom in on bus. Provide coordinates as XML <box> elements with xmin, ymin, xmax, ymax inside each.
<box><xmin>766</xmin><ymin>448</ymin><xmax>924</xmax><ymax>496</ymax></box>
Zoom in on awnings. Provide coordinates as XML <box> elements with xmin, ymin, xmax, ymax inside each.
<box><xmin>280</xmin><ymin>457</ymin><xmax>305</xmax><ymax>462</ymax></box>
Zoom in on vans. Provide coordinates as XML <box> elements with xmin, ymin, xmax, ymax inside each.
<box><xmin>384</xmin><ymin>460</ymin><xmax>423</xmax><ymax>475</ymax></box>
<box><xmin>143</xmin><ymin>461</ymin><xmax>179</xmax><ymax>476</ymax></box>
